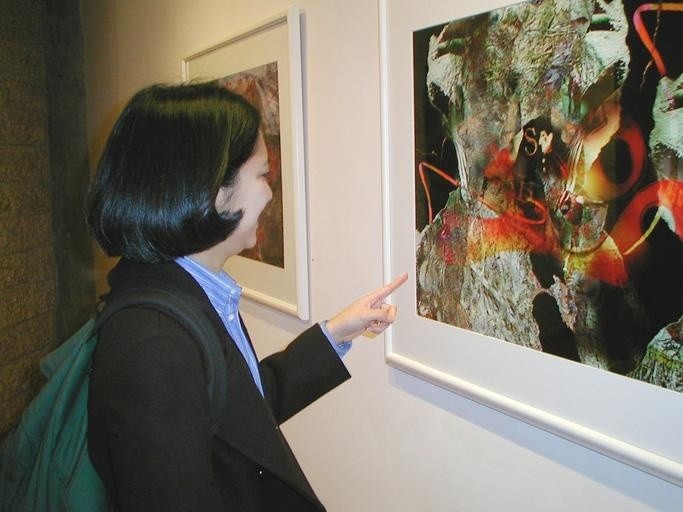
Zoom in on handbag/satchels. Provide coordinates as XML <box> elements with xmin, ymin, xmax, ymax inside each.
<box><xmin>1</xmin><ymin>317</ymin><xmax>110</xmax><ymax>512</ymax></box>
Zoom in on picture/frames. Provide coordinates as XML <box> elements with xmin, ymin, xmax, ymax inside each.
<box><xmin>377</xmin><ymin>1</ymin><xmax>683</xmax><ymax>488</ymax></box>
<box><xmin>180</xmin><ymin>6</ymin><xmax>309</xmax><ymax>322</ymax></box>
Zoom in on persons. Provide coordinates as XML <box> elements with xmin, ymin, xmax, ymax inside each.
<box><xmin>86</xmin><ymin>82</ymin><xmax>409</xmax><ymax>511</ymax></box>
